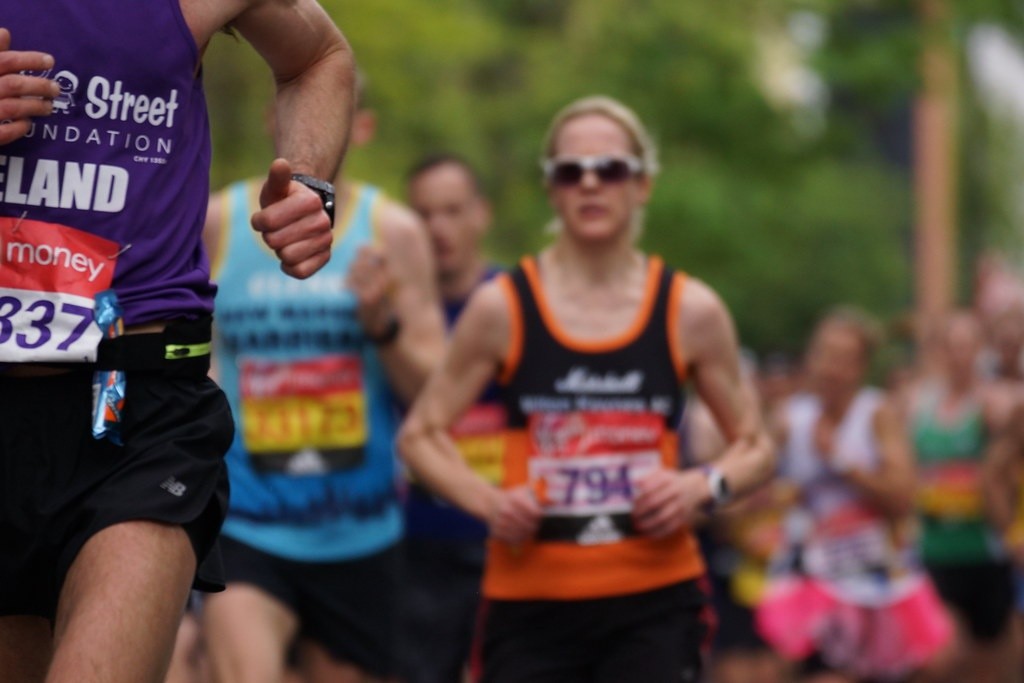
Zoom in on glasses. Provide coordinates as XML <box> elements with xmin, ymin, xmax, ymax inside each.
<box><xmin>546</xmin><ymin>156</ymin><xmax>644</xmax><ymax>183</ymax></box>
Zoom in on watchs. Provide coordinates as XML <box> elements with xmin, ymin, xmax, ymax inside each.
<box><xmin>290</xmin><ymin>173</ymin><xmax>336</xmax><ymax>225</ymax></box>
<box><xmin>699</xmin><ymin>464</ymin><xmax>728</xmax><ymax>513</ymax></box>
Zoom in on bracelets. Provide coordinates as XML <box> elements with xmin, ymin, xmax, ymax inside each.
<box><xmin>367</xmin><ymin>319</ymin><xmax>398</xmax><ymax>344</ymax></box>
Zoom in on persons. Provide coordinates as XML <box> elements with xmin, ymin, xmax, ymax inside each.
<box><xmin>157</xmin><ymin>67</ymin><xmax>1024</xmax><ymax>683</ymax></box>
<box><xmin>0</xmin><ymin>0</ymin><xmax>356</xmax><ymax>683</ymax></box>
